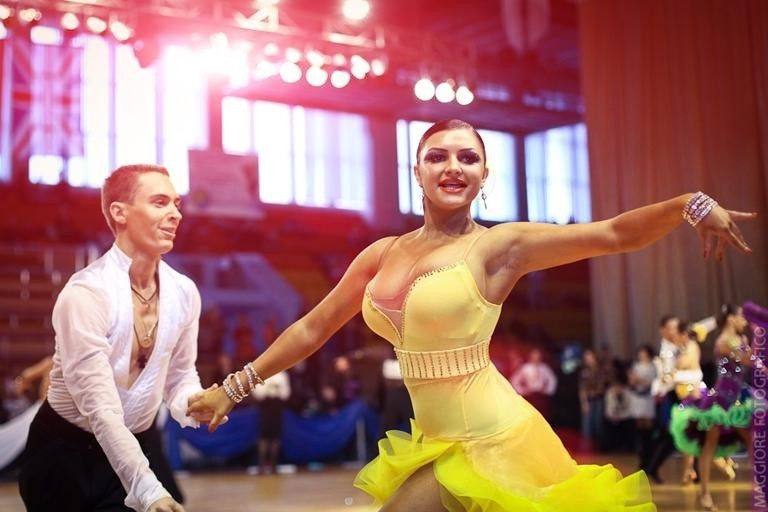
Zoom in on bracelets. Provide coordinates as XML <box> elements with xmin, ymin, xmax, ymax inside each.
<box><xmin>223</xmin><ymin>360</ymin><xmax>264</xmax><ymax>405</ymax></box>
<box><xmin>683</xmin><ymin>190</ymin><xmax>717</xmax><ymax>230</ymax></box>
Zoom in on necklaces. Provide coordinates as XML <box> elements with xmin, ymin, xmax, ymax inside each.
<box><xmin>130</xmin><ymin>282</ymin><xmax>161</xmax><ymax>304</ymax></box>
<box><xmin>133</xmin><ymin>319</ymin><xmax>158</xmax><ymax>368</ymax></box>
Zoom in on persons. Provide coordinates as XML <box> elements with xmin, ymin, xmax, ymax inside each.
<box><xmin>487</xmin><ymin>301</ymin><xmax>768</xmax><ymax>488</ymax></box>
<box><xmin>198</xmin><ymin>304</ymin><xmax>413</xmax><ymax>477</ymax></box>
<box><xmin>15</xmin><ymin>162</ymin><xmax>227</xmax><ymax>512</ymax></box>
<box><xmin>669</xmin><ymin>301</ymin><xmax>767</xmax><ymax>512</ymax></box>
<box><xmin>187</xmin><ymin>116</ymin><xmax>757</xmax><ymax>512</ymax></box>
